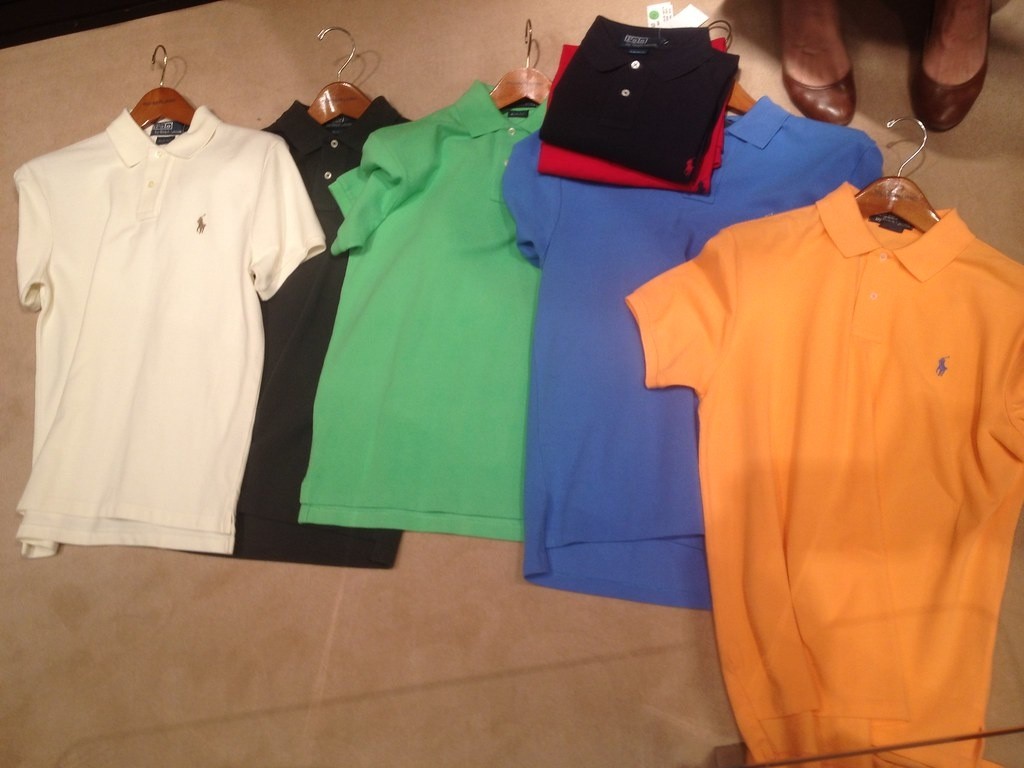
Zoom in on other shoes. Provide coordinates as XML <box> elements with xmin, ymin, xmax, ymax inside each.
<box><xmin>912</xmin><ymin>0</ymin><xmax>992</xmax><ymax>131</ymax></box>
<box><xmin>782</xmin><ymin>65</ymin><xmax>855</xmax><ymax>129</ymax></box>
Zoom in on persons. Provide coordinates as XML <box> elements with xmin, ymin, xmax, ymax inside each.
<box><xmin>775</xmin><ymin>2</ymin><xmax>995</xmax><ymax>135</ymax></box>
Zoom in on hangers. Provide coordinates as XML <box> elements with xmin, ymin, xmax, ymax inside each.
<box><xmin>256</xmin><ymin>27</ymin><xmax>414</xmax><ymax>150</ymax></box>
<box><xmin>363</xmin><ymin>19</ymin><xmax>554</xmax><ymax>152</ymax></box>
<box><xmin>725</xmin><ymin>112</ymin><xmax>1024</xmax><ymax>297</ymax></box>
<box><xmin>15</xmin><ymin>42</ymin><xmax>286</xmax><ymax>182</ymax></box>
<box><xmin>514</xmin><ymin>17</ymin><xmax>871</xmax><ymax>159</ymax></box>
